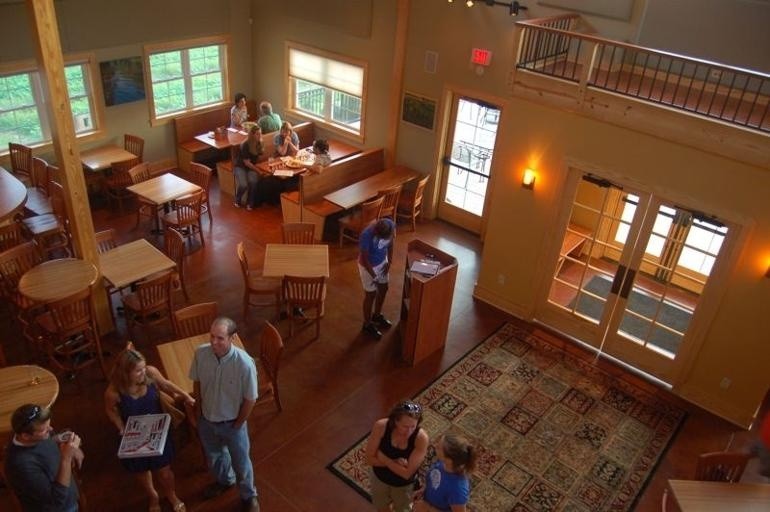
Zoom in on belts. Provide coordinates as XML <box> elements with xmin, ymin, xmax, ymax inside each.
<box><xmin>202</xmin><ymin>416</ymin><xmax>236</xmax><ymax>424</ymax></box>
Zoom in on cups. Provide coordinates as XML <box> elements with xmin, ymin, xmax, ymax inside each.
<box><xmin>58</xmin><ymin>431</ymin><xmax>72</xmax><ymax>441</ymax></box>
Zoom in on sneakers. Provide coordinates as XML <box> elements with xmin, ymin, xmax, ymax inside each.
<box><xmin>363</xmin><ymin>322</ymin><xmax>383</xmax><ymax>340</ymax></box>
<box><xmin>372</xmin><ymin>313</ymin><xmax>392</xmax><ymax>329</ymax></box>
<box><xmin>235</xmin><ymin>202</ymin><xmax>252</xmax><ymax>211</ymax></box>
<box><xmin>202</xmin><ymin>482</ymin><xmax>236</xmax><ymax>498</ymax></box>
<box><xmin>239</xmin><ymin>492</ymin><xmax>260</xmax><ymax>512</ymax></box>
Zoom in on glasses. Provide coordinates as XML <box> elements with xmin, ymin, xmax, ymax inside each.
<box><xmin>20</xmin><ymin>405</ymin><xmax>42</xmax><ymax>431</ymax></box>
<box><xmin>402</xmin><ymin>404</ymin><xmax>421</xmax><ymax>413</ymax></box>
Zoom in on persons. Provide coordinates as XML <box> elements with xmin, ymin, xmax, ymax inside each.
<box><xmin>414</xmin><ymin>431</ymin><xmax>479</xmax><ymax>512</ymax></box>
<box><xmin>357</xmin><ymin>219</ymin><xmax>396</xmax><ymax>339</ymax></box>
<box><xmin>298</xmin><ymin>138</ymin><xmax>330</xmax><ymax>181</ymax></box>
<box><xmin>230</xmin><ymin>93</ymin><xmax>249</xmax><ymax>128</ymax></box>
<box><xmin>4</xmin><ymin>404</ymin><xmax>83</xmax><ymax>511</ymax></box>
<box><xmin>101</xmin><ymin>351</ymin><xmax>196</xmax><ymax>512</ymax></box>
<box><xmin>232</xmin><ymin>125</ymin><xmax>265</xmax><ymax>211</ymax></box>
<box><xmin>189</xmin><ymin>315</ymin><xmax>264</xmax><ymax>512</ymax></box>
<box><xmin>255</xmin><ymin>100</ymin><xmax>282</xmax><ymax>132</ymax></box>
<box><xmin>272</xmin><ymin>121</ymin><xmax>299</xmax><ymax>157</ymax></box>
<box><xmin>366</xmin><ymin>400</ymin><xmax>428</xmax><ymax>511</ymax></box>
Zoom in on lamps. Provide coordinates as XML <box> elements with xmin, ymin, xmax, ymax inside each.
<box><xmin>521</xmin><ymin>168</ymin><xmax>536</xmax><ymax>190</ymax></box>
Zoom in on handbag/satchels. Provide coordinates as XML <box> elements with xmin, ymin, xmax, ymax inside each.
<box><xmin>414</xmin><ymin>478</ymin><xmax>422</xmax><ymax>490</ymax></box>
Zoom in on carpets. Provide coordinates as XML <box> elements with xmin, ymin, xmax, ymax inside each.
<box><xmin>326</xmin><ymin>317</ymin><xmax>690</xmax><ymax>512</ymax></box>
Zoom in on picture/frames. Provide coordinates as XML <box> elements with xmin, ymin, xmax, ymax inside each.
<box><xmin>98</xmin><ymin>55</ymin><xmax>147</xmax><ymax>108</ymax></box>
<box><xmin>399</xmin><ymin>90</ymin><xmax>438</xmax><ymax>132</ymax></box>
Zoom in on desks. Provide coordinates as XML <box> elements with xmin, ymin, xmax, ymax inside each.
<box><xmin>662</xmin><ymin>479</ymin><xmax>769</xmax><ymax>512</ymax></box>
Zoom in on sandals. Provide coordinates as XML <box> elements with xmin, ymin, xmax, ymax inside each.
<box><xmin>149</xmin><ymin>492</ymin><xmax>161</xmax><ymax>511</ymax></box>
<box><xmin>165</xmin><ymin>494</ymin><xmax>186</xmax><ymax>512</ymax></box>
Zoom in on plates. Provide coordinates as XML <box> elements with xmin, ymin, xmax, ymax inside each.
<box><xmin>241</xmin><ymin>121</ymin><xmax>256</xmax><ymax>129</ymax></box>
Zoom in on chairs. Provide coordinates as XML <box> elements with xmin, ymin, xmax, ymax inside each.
<box><xmin>694</xmin><ymin>451</ymin><xmax>749</xmax><ymax>482</ymax></box>
